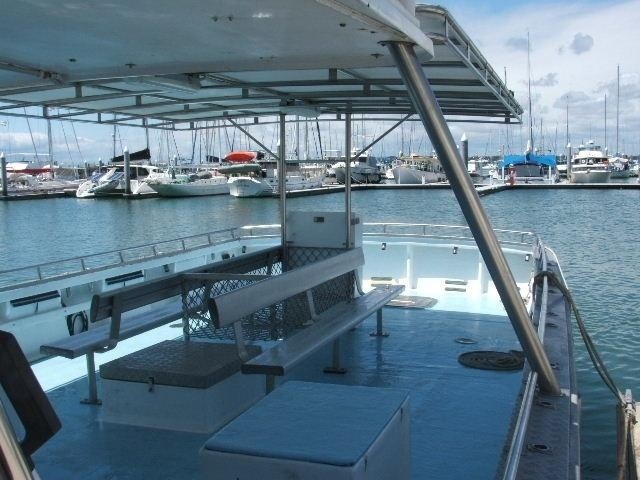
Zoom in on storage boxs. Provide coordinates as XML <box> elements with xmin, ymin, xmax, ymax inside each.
<box><xmin>208</xmin><ymin>246</ymin><xmax>405</xmax><ymax>395</ymax></box>
<box><xmin>40</xmin><ymin>245</ymin><xmax>280</xmax><ymax>405</ymax></box>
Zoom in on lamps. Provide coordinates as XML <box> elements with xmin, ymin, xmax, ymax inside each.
<box><xmin>99</xmin><ymin>272</ymin><xmax>271</xmax><ymax>392</ymax></box>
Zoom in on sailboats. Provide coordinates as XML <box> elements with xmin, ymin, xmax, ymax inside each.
<box><xmin>2</xmin><ymin>104</ymin><xmax>89</xmax><ymax>199</ymax></box>
<box><xmin>218</xmin><ymin>115</ymin><xmax>328</xmax><ymax>199</ymax></box>
<box><xmin>603</xmin><ymin>64</ymin><xmax>639</xmax><ymax>179</ymax></box>
<box><xmin>496</xmin><ymin>30</ymin><xmax>561</xmax><ymax>185</ymax></box>
<box><xmin>469</xmin><ymin>66</ymin><xmax>568</xmax><ymax>185</ymax></box>
<box><xmin>565</xmin><ymin>93</ymin><xmax>611</xmax><ymax>183</ymax></box>
<box><xmin>75</xmin><ymin>113</ymin><xmax>187</xmax><ymax>198</ymax></box>
<box><xmin>145</xmin><ymin>119</ymin><xmax>230</xmax><ymax>197</ymax></box>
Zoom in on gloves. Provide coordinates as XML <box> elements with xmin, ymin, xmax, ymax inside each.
<box><xmin>1</xmin><ymin>1</ymin><xmax>639</xmax><ymax>479</ymax></box>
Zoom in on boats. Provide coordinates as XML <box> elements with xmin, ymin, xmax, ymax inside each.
<box><xmin>1</xmin><ymin>1</ymin><xmax>639</xmax><ymax>479</ymax></box>
<box><xmin>391</xmin><ymin>114</ymin><xmax>448</xmax><ymax>184</ymax></box>
<box><xmin>331</xmin><ymin>114</ymin><xmax>387</xmax><ymax>185</ymax></box>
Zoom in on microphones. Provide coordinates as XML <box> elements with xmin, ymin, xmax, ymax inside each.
<box><xmin>197</xmin><ymin>380</ymin><xmax>412</xmax><ymax>480</ymax></box>
